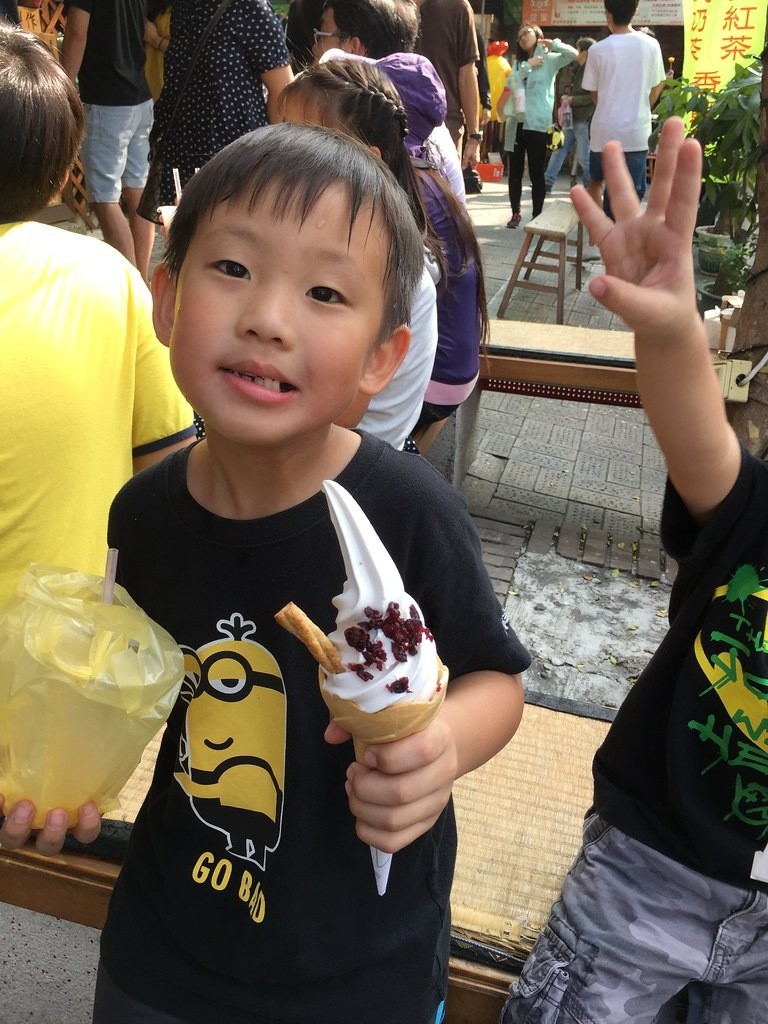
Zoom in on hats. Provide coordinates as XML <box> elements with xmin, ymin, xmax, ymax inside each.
<box><xmin>317</xmin><ymin>47</ymin><xmax>449</xmax><ymax>157</ymax></box>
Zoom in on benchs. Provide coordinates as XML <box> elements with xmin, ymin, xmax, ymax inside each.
<box><xmin>495</xmin><ymin>198</ymin><xmax>589</xmax><ymax>326</ymax></box>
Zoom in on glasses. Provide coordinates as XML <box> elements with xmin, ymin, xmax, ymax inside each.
<box><xmin>516</xmin><ymin>29</ymin><xmax>535</xmax><ymax>42</ymax></box>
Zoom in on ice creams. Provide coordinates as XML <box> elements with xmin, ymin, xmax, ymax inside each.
<box><xmin>273</xmin><ymin>480</ymin><xmax>449</xmax><ymax>897</ymax></box>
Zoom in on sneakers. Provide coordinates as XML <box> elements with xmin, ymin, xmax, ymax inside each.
<box><xmin>506</xmin><ymin>212</ymin><xmax>523</xmax><ymax>229</ymax></box>
<box><xmin>574</xmin><ymin>242</ymin><xmax>602</xmax><ymax>263</ymax></box>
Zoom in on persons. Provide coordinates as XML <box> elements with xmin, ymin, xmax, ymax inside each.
<box><xmin>462</xmin><ymin>30</ymin><xmax>492</xmax><ymax>159</ymax></box>
<box><xmin>143</xmin><ymin>0</ymin><xmax>170</xmax><ymax>101</ymax></box>
<box><xmin>155</xmin><ymin>60</ymin><xmax>437</xmax><ymax>455</ymax></box>
<box><xmin>406</xmin><ymin>1</ymin><xmax>484</xmax><ymax>171</ymax></box>
<box><xmin>503</xmin><ymin>114</ymin><xmax>768</xmax><ymax>1023</ymax></box>
<box><xmin>529</xmin><ymin>38</ymin><xmax>597</xmax><ymax>194</ymax></box>
<box><xmin>1</xmin><ymin>28</ymin><xmax>193</xmax><ymax>828</ymax></box>
<box><xmin>59</xmin><ymin>0</ymin><xmax>153</xmax><ymax>288</ymax></box>
<box><xmin>313</xmin><ymin>0</ymin><xmax>422</xmax><ymax>64</ymax></box>
<box><xmin>373</xmin><ymin>54</ymin><xmax>489</xmax><ymax>426</ymax></box>
<box><xmin>497</xmin><ymin>20</ymin><xmax>579</xmax><ymax>228</ymax></box>
<box><xmin>2</xmin><ymin>125</ymin><xmax>533</xmax><ymax>1024</ymax></box>
<box><xmin>581</xmin><ymin>1</ymin><xmax>666</xmax><ymax>223</ymax></box>
<box><xmin>147</xmin><ymin>1</ymin><xmax>294</xmax><ymax>207</ymax></box>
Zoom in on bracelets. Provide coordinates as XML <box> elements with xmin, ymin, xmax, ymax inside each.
<box><xmin>159</xmin><ymin>38</ymin><xmax>165</xmax><ymax>49</ymax></box>
<box><xmin>467</xmin><ymin>131</ymin><xmax>484</xmax><ymax>142</ymax></box>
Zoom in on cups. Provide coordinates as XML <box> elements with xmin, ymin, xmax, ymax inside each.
<box><xmin>514</xmin><ymin>88</ymin><xmax>525</xmax><ymax>113</ymax></box>
<box><xmin>156</xmin><ymin>205</ymin><xmax>178</xmax><ymax>237</ymax></box>
<box><xmin>563</xmin><ymin>111</ymin><xmax>571</xmax><ymax>128</ymax></box>
<box><xmin>0</xmin><ymin>600</ymin><xmax>184</xmax><ymax>831</ymax></box>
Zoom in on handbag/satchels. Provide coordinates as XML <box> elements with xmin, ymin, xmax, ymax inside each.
<box><xmin>134</xmin><ymin>159</ymin><xmax>165</xmax><ymax>225</ymax></box>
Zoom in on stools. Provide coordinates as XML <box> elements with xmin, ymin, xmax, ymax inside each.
<box><xmin>459</xmin><ymin>312</ymin><xmax>657</xmax><ymax>516</ymax></box>
